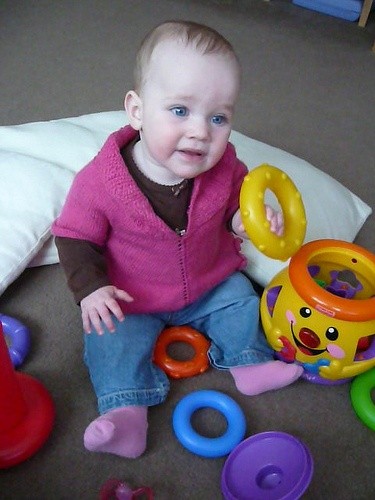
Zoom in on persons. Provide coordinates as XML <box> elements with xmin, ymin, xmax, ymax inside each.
<box><xmin>51</xmin><ymin>20</ymin><xmax>305</xmax><ymax>459</ymax></box>
